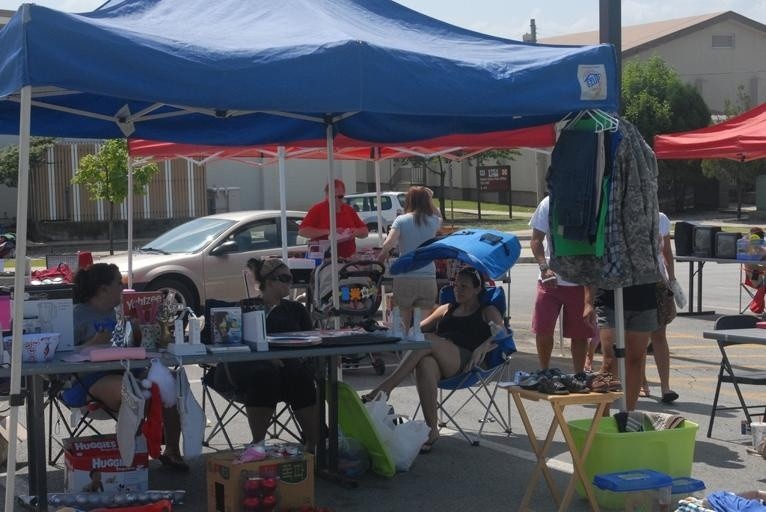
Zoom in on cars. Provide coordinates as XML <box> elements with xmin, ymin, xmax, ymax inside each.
<box><xmin>94</xmin><ymin>210</ymin><xmax>385</xmax><ymax>333</ymax></box>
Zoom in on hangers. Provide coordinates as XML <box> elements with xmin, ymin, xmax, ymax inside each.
<box><xmin>555</xmin><ymin>108</ymin><xmax>620</xmax><ymax>134</ymax></box>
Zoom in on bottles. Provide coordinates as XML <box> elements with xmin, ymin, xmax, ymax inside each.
<box><xmin>489</xmin><ymin>320</ymin><xmax>509</xmax><ymax>338</ymax></box>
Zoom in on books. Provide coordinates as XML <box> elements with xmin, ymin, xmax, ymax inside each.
<box><xmin>209</xmin><ymin>306</ymin><xmax>244</xmax><ymax>345</ymax></box>
<box><xmin>205</xmin><ymin>343</ymin><xmax>251</xmax><ymax>355</ymax></box>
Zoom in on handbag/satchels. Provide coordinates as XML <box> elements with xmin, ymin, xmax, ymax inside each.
<box><xmin>652</xmin><ymin>284</ymin><xmax>678</xmax><ymax>329</ymax></box>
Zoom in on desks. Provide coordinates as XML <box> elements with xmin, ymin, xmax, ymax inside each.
<box><xmin>503</xmin><ymin>379</ymin><xmax>625</xmax><ymax>512</ymax></box>
<box><xmin>0</xmin><ymin>333</ymin><xmax>430</xmax><ymax>512</ymax></box>
<box><xmin>703</xmin><ymin>328</ymin><xmax>766</xmax><ymax>346</ymax></box>
<box><xmin>673</xmin><ymin>254</ymin><xmax>765</xmax><ymax>322</ymax></box>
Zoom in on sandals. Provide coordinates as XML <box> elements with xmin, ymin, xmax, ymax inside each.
<box><xmin>661</xmin><ymin>390</ymin><xmax>679</xmax><ymax>402</ymax></box>
<box><xmin>420</xmin><ymin>431</ymin><xmax>440</xmax><ymax>454</ymax></box>
<box><xmin>639</xmin><ymin>381</ymin><xmax>650</xmax><ymax>397</ymax></box>
<box><xmin>362</xmin><ymin>389</ymin><xmax>390</xmax><ymax>403</ymax></box>
<box><xmin>520</xmin><ymin>368</ymin><xmax>550</xmax><ymax>390</ymax></box>
<box><xmin>159</xmin><ymin>451</ymin><xmax>191</xmax><ymax>472</ymax></box>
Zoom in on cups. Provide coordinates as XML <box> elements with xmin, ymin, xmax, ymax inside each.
<box><xmin>750</xmin><ymin>421</ymin><xmax>765</xmax><ymax>452</ymax></box>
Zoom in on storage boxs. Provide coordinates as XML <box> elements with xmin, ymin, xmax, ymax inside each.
<box><xmin>204</xmin><ymin>446</ymin><xmax>315</xmax><ymax>512</ymax></box>
<box><xmin>59</xmin><ymin>432</ymin><xmax>150</xmax><ymax>494</ymax></box>
<box><xmin>567</xmin><ymin>411</ymin><xmax>766</xmax><ymax>512</ymax></box>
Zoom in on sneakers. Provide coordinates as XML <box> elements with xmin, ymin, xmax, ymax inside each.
<box><xmin>581</xmin><ymin>372</ymin><xmax>608</xmax><ymax>393</ymax></box>
<box><xmin>559</xmin><ymin>374</ymin><xmax>590</xmax><ymax>394</ymax></box>
<box><xmin>537</xmin><ymin>376</ymin><xmax>569</xmax><ymax>395</ymax></box>
<box><xmin>600</xmin><ymin>373</ymin><xmax>623</xmax><ymax>391</ymax></box>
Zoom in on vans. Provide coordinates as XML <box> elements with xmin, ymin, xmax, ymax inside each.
<box><xmin>343</xmin><ymin>189</ymin><xmax>408</xmax><ymax>233</ymax></box>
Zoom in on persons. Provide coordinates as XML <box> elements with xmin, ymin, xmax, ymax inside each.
<box><xmin>635</xmin><ymin>211</ymin><xmax>682</xmax><ymax>404</ymax></box>
<box><xmin>211</xmin><ymin>255</ymin><xmax>323</xmax><ymax>452</ymax></box>
<box><xmin>298</xmin><ymin>180</ymin><xmax>370</xmax><ymax>261</ymax></box>
<box><xmin>590</xmin><ymin>284</ymin><xmax>661</xmax><ymax>418</ymax></box>
<box><xmin>749</xmin><ymin>227</ymin><xmax>766</xmax><ymax>241</ymax></box>
<box><xmin>361</xmin><ymin>264</ymin><xmax>508</xmax><ymax>456</ymax></box>
<box><xmin>59</xmin><ymin>262</ymin><xmax>191</xmax><ymax>474</ymax></box>
<box><xmin>374</xmin><ymin>184</ymin><xmax>446</xmax><ymax>334</ymax></box>
<box><xmin>525</xmin><ymin>195</ymin><xmax>596</xmax><ymax>375</ymax></box>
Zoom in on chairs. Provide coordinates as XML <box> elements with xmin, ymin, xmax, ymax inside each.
<box><xmin>195</xmin><ymin>297</ymin><xmax>306</xmax><ymax>455</ymax></box>
<box><xmin>411</xmin><ymin>283</ymin><xmax>516</xmax><ymax>447</ymax></box>
<box><xmin>740</xmin><ymin>261</ymin><xmax>765</xmax><ymax>318</ymax></box>
<box><xmin>45</xmin><ymin>371</ymin><xmax>157</xmax><ymax>463</ymax></box>
<box><xmin>708</xmin><ymin>314</ymin><xmax>766</xmax><ymax>438</ymax></box>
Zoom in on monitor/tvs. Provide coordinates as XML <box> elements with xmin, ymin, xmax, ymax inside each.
<box><xmin>691</xmin><ymin>225</ymin><xmax>721</xmax><ymax>258</ymax></box>
<box><xmin>715</xmin><ymin>232</ymin><xmax>743</xmax><ymax>259</ymax></box>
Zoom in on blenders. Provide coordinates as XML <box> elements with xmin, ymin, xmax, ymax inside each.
<box><xmin>37</xmin><ymin>301</ymin><xmax>58</xmax><ymax>332</ymax></box>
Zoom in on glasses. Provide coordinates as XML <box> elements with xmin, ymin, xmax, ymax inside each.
<box><xmin>334</xmin><ymin>194</ymin><xmax>344</xmax><ymax>199</ymax></box>
<box><xmin>271</xmin><ymin>274</ymin><xmax>292</xmax><ymax>283</ymax></box>
<box><xmin>466</xmin><ymin>267</ymin><xmax>482</xmax><ymax>287</ymax></box>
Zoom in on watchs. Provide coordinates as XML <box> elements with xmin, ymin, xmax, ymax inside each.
<box><xmin>539</xmin><ymin>263</ymin><xmax>549</xmax><ymax>272</ymax></box>
